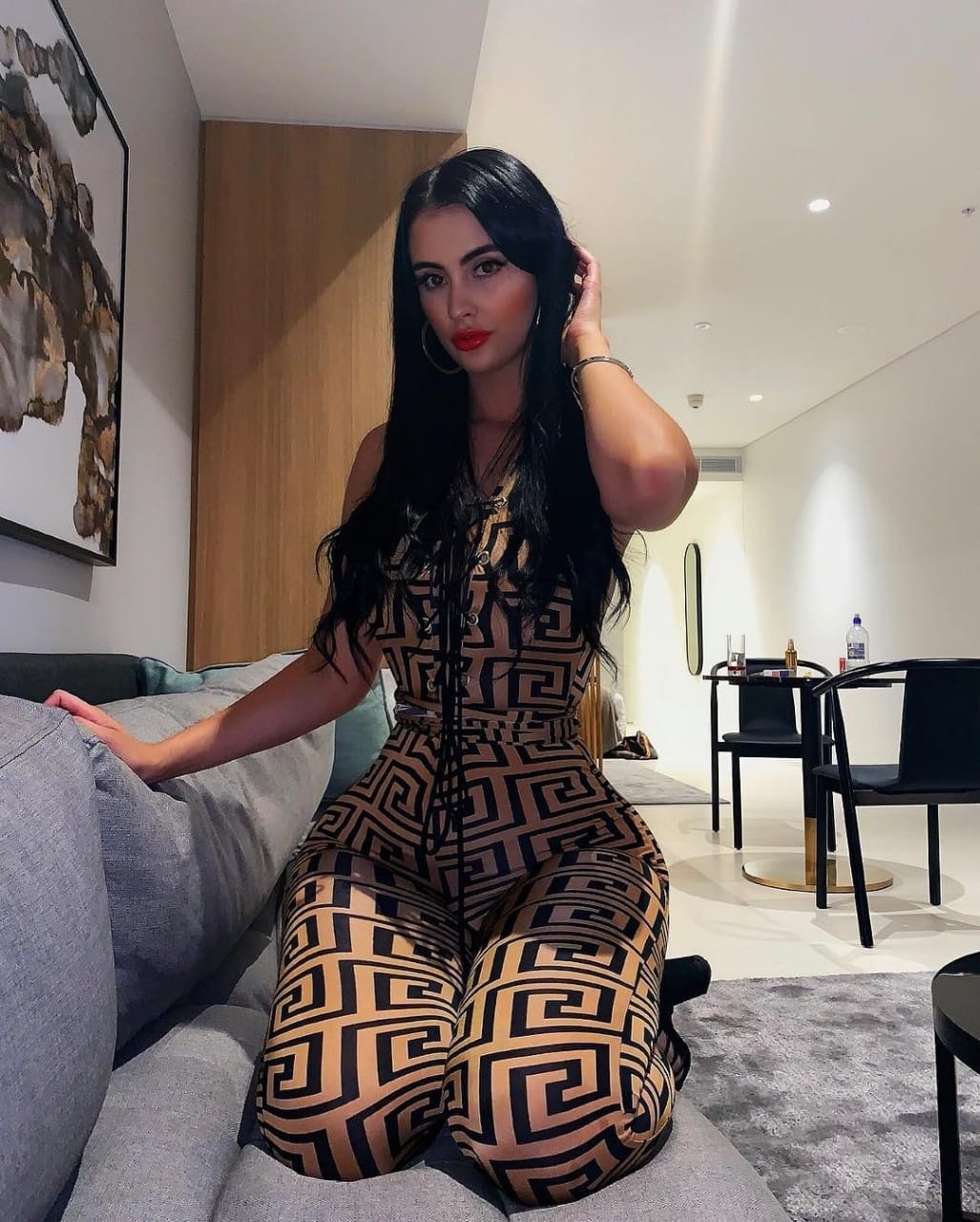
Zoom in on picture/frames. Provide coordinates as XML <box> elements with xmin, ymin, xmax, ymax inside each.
<box><xmin>0</xmin><ymin>0</ymin><xmax>131</xmax><ymax>567</ymax></box>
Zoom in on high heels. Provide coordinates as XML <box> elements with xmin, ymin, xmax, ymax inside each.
<box><xmin>655</xmin><ymin>955</ymin><xmax>712</xmax><ymax>1091</ymax></box>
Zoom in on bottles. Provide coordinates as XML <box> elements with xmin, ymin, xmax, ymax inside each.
<box><xmin>846</xmin><ymin>613</ymin><xmax>871</xmax><ymax>674</ymax></box>
<box><xmin>785</xmin><ymin>638</ymin><xmax>798</xmax><ymax>671</ymax></box>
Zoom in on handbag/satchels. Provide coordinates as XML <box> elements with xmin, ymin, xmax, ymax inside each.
<box><xmin>601</xmin><ymin>730</ymin><xmax>657</xmax><ymax>759</ymax></box>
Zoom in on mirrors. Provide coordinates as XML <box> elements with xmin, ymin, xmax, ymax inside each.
<box><xmin>686</xmin><ymin>543</ymin><xmax>704</xmax><ymax>675</ymax></box>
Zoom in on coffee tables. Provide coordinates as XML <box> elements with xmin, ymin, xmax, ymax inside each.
<box><xmin>931</xmin><ymin>952</ymin><xmax>980</xmax><ymax>1222</ymax></box>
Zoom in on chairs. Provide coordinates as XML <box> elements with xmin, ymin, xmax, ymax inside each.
<box><xmin>813</xmin><ymin>657</ymin><xmax>980</xmax><ymax>949</ymax></box>
<box><xmin>711</xmin><ymin>657</ymin><xmax>838</xmax><ymax>849</ymax></box>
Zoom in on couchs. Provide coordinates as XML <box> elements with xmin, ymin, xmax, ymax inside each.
<box><xmin>0</xmin><ymin>642</ymin><xmax>786</xmax><ymax>1222</ymax></box>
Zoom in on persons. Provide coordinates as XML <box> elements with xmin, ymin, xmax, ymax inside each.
<box><xmin>44</xmin><ymin>148</ymin><xmax>711</xmax><ymax>1210</ymax></box>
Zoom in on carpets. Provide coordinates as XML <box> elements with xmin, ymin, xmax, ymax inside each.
<box><xmin>590</xmin><ymin>747</ymin><xmax>733</xmax><ymax>806</ymax></box>
<box><xmin>675</xmin><ymin>973</ymin><xmax>980</xmax><ymax>1222</ymax></box>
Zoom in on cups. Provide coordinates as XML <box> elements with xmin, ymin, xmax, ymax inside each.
<box><xmin>726</xmin><ymin>634</ymin><xmax>747</xmax><ymax>677</ymax></box>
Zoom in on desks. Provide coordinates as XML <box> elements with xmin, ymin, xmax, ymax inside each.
<box><xmin>699</xmin><ymin>671</ymin><xmax>890</xmax><ymax>893</ymax></box>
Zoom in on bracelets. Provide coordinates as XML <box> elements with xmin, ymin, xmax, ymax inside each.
<box><xmin>571</xmin><ymin>355</ymin><xmax>633</xmax><ymax>395</ymax></box>
